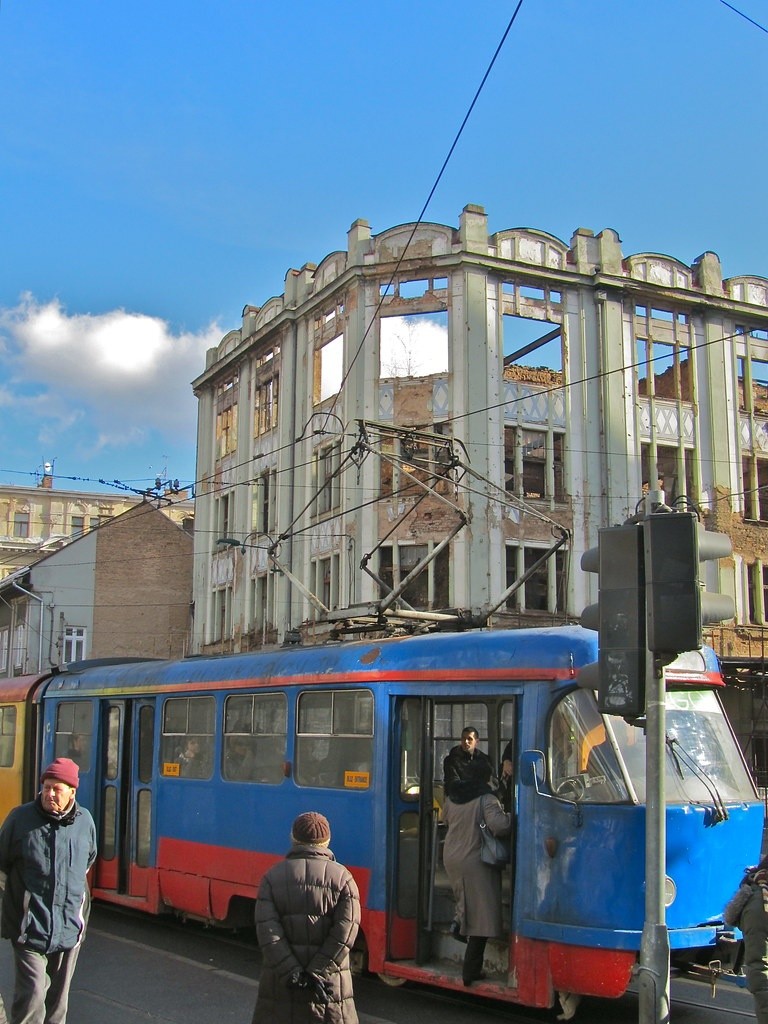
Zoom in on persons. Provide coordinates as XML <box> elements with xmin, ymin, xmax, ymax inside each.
<box><xmin>252</xmin><ymin>812</ymin><xmax>361</xmax><ymax>1024</ymax></box>
<box><xmin>443</xmin><ymin>758</ymin><xmax>513</xmax><ymax>987</ymax></box>
<box><xmin>500</xmin><ymin>714</ymin><xmax>572</xmax><ymax>839</ymax></box>
<box><xmin>63</xmin><ymin>734</ymin><xmax>90</xmax><ymax>771</ymax></box>
<box><xmin>173</xmin><ymin>730</ymin><xmax>373</xmax><ymax>788</ymax></box>
<box><xmin>723</xmin><ymin>854</ymin><xmax>768</xmax><ymax>1024</ymax></box>
<box><xmin>0</xmin><ymin>759</ymin><xmax>97</xmax><ymax>1024</ymax></box>
<box><xmin>444</xmin><ymin>726</ymin><xmax>492</xmax><ymax>797</ymax></box>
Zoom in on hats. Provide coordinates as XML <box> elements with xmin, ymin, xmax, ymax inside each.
<box><xmin>41</xmin><ymin>757</ymin><xmax>79</xmax><ymax>788</ymax></box>
<box><xmin>758</xmin><ymin>855</ymin><xmax>768</xmax><ymax>868</ymax></box>
<box><xmin>291</xmin><ymin>812</ymin><xmax>330</xmax><ymax>847</ymax></box>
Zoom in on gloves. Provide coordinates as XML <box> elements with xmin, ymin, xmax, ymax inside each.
<box><xmin>297</xmin><ymin>972</ymin><xmax>334</xmax><ymax>1003</ymax></box>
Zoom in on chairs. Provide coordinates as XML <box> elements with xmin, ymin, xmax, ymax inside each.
<box><xmin>319</xmin><ymin>729</ymin><xmax>368</xmax><ymax>790</ymax></box>
<box><xmin>401</xmin><ymin>785</ymin><xmax>446</xmax><ymax>826</ymax></box>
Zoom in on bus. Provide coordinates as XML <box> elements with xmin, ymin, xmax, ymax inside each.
<box><xmin>1</xmin><ymin>419</ymin><xmax>768</xmax><ymax>1024</ymax></box>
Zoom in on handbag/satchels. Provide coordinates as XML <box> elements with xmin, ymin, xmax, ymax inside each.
<box><xmin>478</xmin><ymin>794</ymin><xmax>512</xmax><ymax>867</ymax></box>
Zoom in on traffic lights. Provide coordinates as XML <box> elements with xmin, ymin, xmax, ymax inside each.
<box><xmin>641</xmin><ymin>508</ymin><xmax>737</xmax><ymax>659</ymax></box>
<box><xmin>575</xmin><ymin>522</ymin><xmax>647</xmax><ymax>722</ymax></box>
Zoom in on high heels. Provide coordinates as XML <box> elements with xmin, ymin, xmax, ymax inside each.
<box><xmin>463</xmin><ymin>972</ymin><xmax>486</xmax><ymax>987</ymax></box>
<box><xmin>449</xmin><ymin>921</ymin><xmax>467</xmax><ymax>943</ymax></box>
